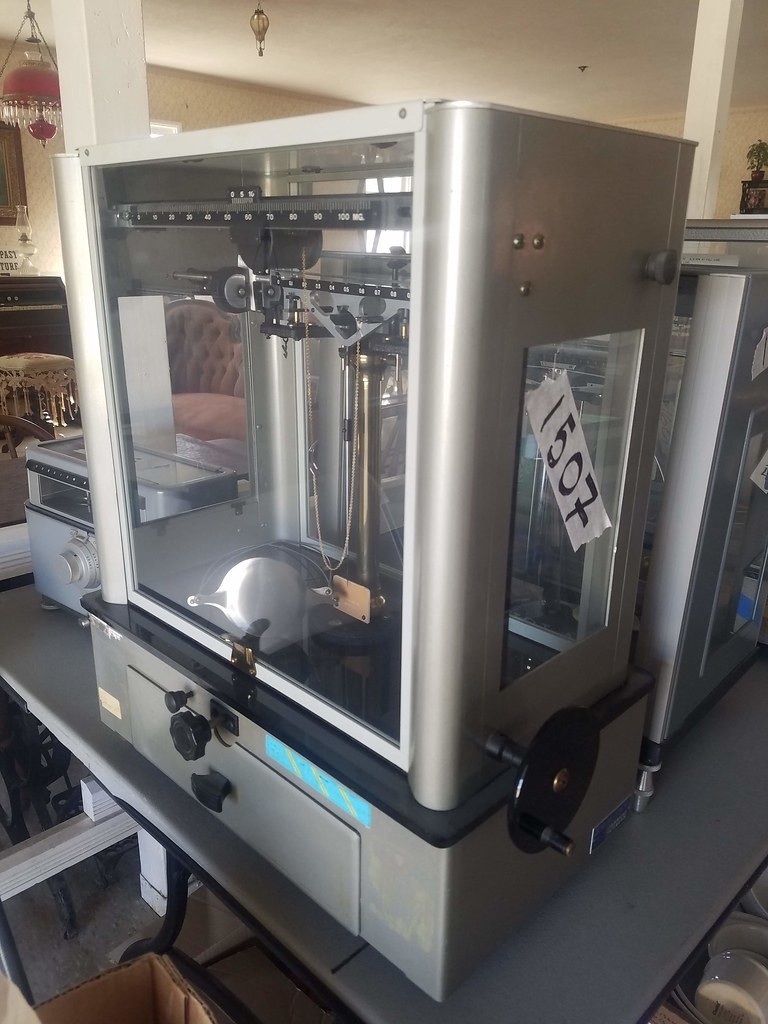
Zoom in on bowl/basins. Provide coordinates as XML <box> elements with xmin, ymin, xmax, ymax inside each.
<box><xmin>671</xmin><ymin>867</ymin><xmax>768</xmax><ymax>1024</ymax></box>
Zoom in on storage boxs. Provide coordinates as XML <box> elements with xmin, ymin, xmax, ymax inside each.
<box><xmin>31</xmin><ymin>951</ymin><xmax>218</xmax><ymax>1024</ymax></box>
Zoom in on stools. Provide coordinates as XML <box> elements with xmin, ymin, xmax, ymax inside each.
<box><xmin>0</xmin><ymin>351</ymin><xmax>77</xmax><ymax>450</ymax></box>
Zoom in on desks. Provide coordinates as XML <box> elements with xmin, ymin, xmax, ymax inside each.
<box><xmin>0</xmin><ymin>565</ymin><xmax>768</xmax><ymax>1023</ymax></box>
